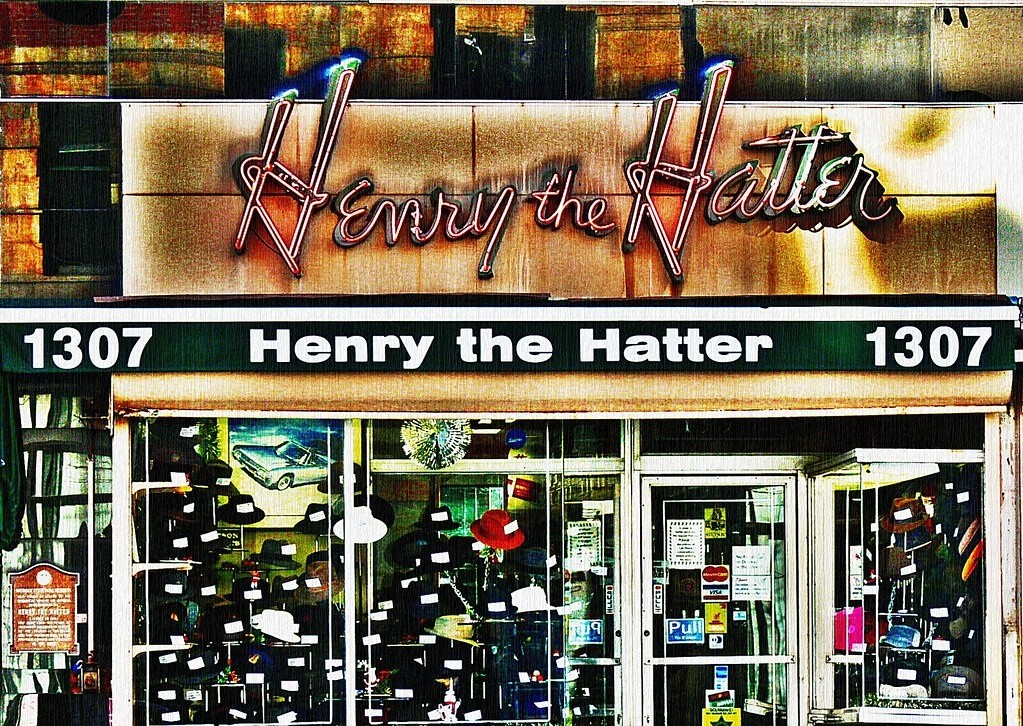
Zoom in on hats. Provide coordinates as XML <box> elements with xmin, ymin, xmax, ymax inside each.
<box><xmin>880</xmin><ymin>498</ymin><xmax>929</xmax><ymax>534</ymax></box>
<box><xmin>413</xmin><ymin>506</ymin><xmax>462</xmax><ymax>531</ymax></box>
<box><xmin>470</xmin><ymin>510</ymin><xmax>525</xmax><ymax>551</ymax></box>
<box><xmin>382</xmin><ymin>532</ymin><xmax>985</xmax><ymax>720</ymax></box>
<box><xmin>135</xmin><ymin>459</ymin><xmax>388</xmax><ymax>723</ymax></box>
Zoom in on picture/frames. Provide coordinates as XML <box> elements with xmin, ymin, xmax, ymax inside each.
<box><xmin>216</xmin><ymin>417</ymin><xmax>361</xmax><ymax>528</ymax></box>
